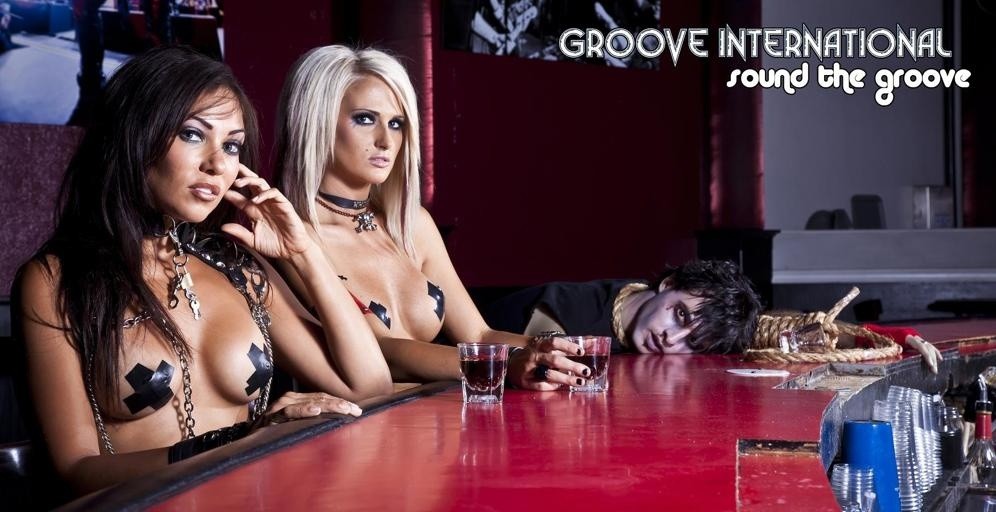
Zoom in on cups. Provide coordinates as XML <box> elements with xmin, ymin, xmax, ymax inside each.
<box><xmin>564</xmin><ymin>334</ymin><xmax>612</xmax><ymax>393</ymax></box>
<box><xmin>830</xmin><ymin>383</ymin><xmax>961</xmax><ymax>512</ymax></box>
<box><xmin>457</xmin><ymin>342</ymin><xmax>509</xmax><ymax>406</ymax></box>
<box><xmin>778</xmin><ymin>319</ymin><xmax>829</xmax><ymax>355</ymax></box>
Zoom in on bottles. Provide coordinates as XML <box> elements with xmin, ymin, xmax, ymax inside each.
<box><xmin>962</xmin><ymin>399</ymin><xmax>994</xmax><ymax>489</ymax></box>
